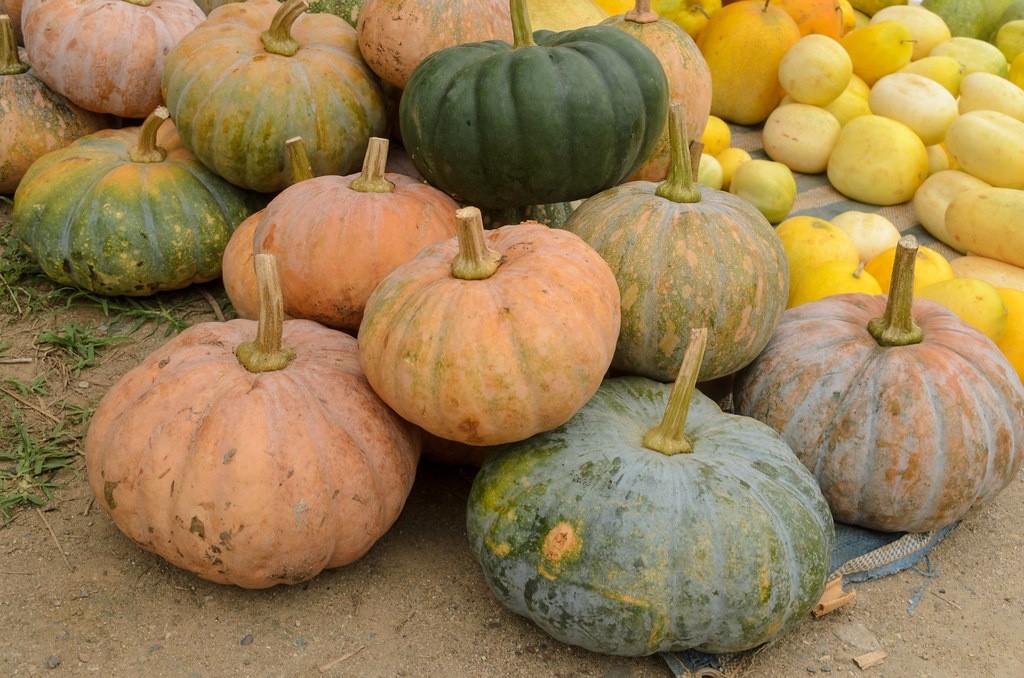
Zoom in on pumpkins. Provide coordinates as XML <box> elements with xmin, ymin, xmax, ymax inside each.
<box><xmin>352</xmin><ymin>206</ymin><xmax>623</xmax><ymax>448</ymax></box>
<box><xmin>466</xmin><ymin>328</ymin><xmax>840</xmax><ymax>655</ymax></box>
<box><xmin>358</xmin><ymin>0</ymin><xmax>518</xmax><ymax>90</ymax></box>
<box><xmin>12</xmin><ymin>107</ymin><xmax>266</xmax><ymax>296</ymax></box>
<box><xmin>596</xmin><ymin>0</ymin><xmax>714</xmax><ymax>186</ymax></box>
<box><xmin>731</xmin><ymin>232</ymin><xmax>1024</xmax><ymax>536</ymax></box>
<box><xmin>254</xmin><ymin>137</ymin><xmax>466</xmax><ymax>331</ymax></box>
<box><xmin>398</xmin><ymin>0</ymin><xmax>671</xmax><ymax>207</ymax></box>
<box><xmin>78</xmin><ymin>250</ymin><xmax>425</xmax><ymax>592</ymax></box>
<box><xmin>1</xmin><ymin>0</ymin><xmax>209</xmax><ymax>195</ymax></box>
<box><xmin>158</xmin><ymin>0</ymin><xmax>387</xmax><ymax>192</ymax></box>
<box><xmin>220</xmin><ymin>206</ymin><xmax>298</xmax><ymax>320</ymax></box>
<box><xmin>559</xmin><ymin>103</ymin><xmax>792</xmax><ymax>385</ymax></box>
